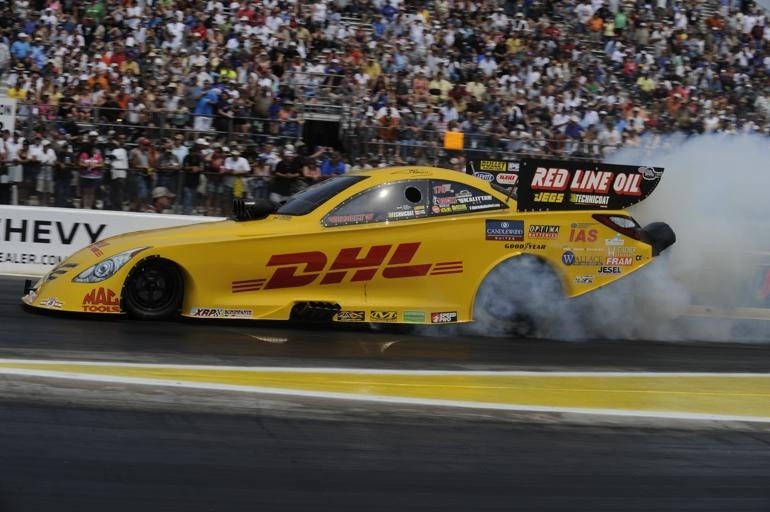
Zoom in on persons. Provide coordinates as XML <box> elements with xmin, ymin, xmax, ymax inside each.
<box><xmin>1</xmin><ymin>2</ymin><xmax>769</xmax><ymax>213</ymax></box>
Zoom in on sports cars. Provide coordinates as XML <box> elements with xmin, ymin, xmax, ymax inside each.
<box><xmin>20</xmin><ymin>157</ymin><xmax>677</xmax><ymax>334</ymax></box>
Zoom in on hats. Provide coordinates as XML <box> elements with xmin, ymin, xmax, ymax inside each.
<box><xmin>14</xmin><ymin>62</ymin><xmax>27</xmax><ymax>70</ymax></box>
<box><xmin>151</xmin><ymin>186</ymin><xmax>176</xmax><ymax>199</ymax></box>
<box><xmin>194</xmin><ymin>138</ymin><xmax>211</xmax><ymax>147</ymax></box>
<box><xmin>222</xmin><ymin>141</ymin><xmax>242</xmax><ymax>156</ymax></box>
<box><xmin>287</xmin><ymin>41</ymin><xmax>298</xmax><ymax>49</ymax></box>
<box><xmin>283</xmin><ymin>150</ymin><xmax>299</xmax><ymax>157</ymax></box>
<box><xmin>17</xmin><ymin>32</ymin><xmax>30</xmax><ymax>38</ymax></box>
<box><xmin>137</xmin><ymin>137</ymin><xmax>152</xmax><ymax>145</ymax></box>
<box><xmin>240</xmin><ymin>15</ymin><xmax>250</xmax><ymax>22</ymax></box>
<box><xmin>40</xmin><ymin>139</ymin><xmax>52</xmax><ymax>147</ymax></box>
<box><xmin>174</xmin><ymin>134</ymin><xmax>185</xmax><ymax>140</ymax></box>
